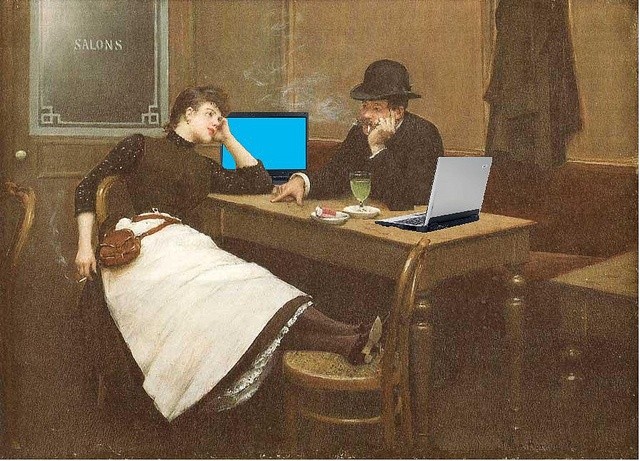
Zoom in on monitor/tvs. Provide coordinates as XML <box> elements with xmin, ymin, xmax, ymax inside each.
<box><xmin>220</xmin><ymin>111</ymin><xmax>309</xmax><ymax>180</ymax></box>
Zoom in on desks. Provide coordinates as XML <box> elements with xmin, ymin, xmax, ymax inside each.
<box><xmin>202</xmin><ymin>182</ymin><xmax>539</xmax><ymax>461</ymax></box>
<box><xmin>550</xmin><ymin>251</ymin><xmax>639</xmax><ymax>460</ymax></box>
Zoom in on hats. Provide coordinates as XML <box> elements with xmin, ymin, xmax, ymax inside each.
<box><xmin>350</xmin><ymin>59</ymin><xmax>422</xmax><ymax>100</ymax></box>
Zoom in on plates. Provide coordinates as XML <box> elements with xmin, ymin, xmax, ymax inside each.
<box><xmin>344</xmin><ymin>205</ymin><xmax>381</xmax><ymax>219</ymax></box>
<box><xmin>309</xmin><ymin>212</ymin><xmax>349</xmax><ymax>226</ymax></box>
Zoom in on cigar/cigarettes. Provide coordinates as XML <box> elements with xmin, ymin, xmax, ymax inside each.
<box><xmin>368</xmin><ymin>124</ymin><xmax>371</xmax><ymax>132</ymax></box>
<box><xmin>79</xmin><ymin>275</ymin><xmax>87</xmax><ymax>282</ymax></box>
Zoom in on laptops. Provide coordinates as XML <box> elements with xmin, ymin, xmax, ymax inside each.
<box><xmin>374</xmin><ymin>157</ymin><xmax>494</xmax><ymax>233</ymax></box>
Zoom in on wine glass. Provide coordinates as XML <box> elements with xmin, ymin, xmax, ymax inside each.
<box><xmin>348</xmin><ymin>170</ymin><xmax>371</xmax><ymax>211</ymax></box>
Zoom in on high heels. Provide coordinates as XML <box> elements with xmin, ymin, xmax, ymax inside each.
<box><xmin>357</xmin><ymin>313</ymin><xmax>390</xmax><ymax>332</ymax></box>
<box><xmin>348</xmin><ymin>316</ymin><xmax>382</xmax><ymax>364</ymax></box>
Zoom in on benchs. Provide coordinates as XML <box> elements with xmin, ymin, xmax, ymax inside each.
<box><xmin>307</xmin><ymin>138</ymin><xmax>638</xmax><ymax>321</ymax></box>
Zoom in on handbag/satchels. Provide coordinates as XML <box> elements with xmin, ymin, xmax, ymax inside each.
<box><xmin>99</xmin><ymin>215</ymin><xmax>180</xmax><ymax>267</ymax></box>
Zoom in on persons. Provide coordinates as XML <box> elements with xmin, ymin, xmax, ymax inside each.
<box><xmin>272</xmin><ymin>59</ymin><xmax>442</xmax><ymax>206</ymax></box>
<box><xmin>73</xmin><ymin>86</ymin><xmax>388</xmax><ymax>365</ymax></box>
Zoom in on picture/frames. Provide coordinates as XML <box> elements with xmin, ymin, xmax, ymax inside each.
<box><xmin>30</xmin><ymin>1</ymin><xmax>169</xmax><ymax>137</ymax></box>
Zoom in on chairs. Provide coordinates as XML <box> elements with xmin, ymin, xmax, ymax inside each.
<box><xmin>96</xmin><ymin>174</ymin><xmax>136</xmax><ymax>411</ymax></box>
<box><xmin>0</xmin><ymin>181</ymin><xmax>35</xmax><ymax>449</ymax></box>
<box><xmin>282</xmin><ymin>239</ymin><xmax>432</xmax><ymax>457</ymax></box>
